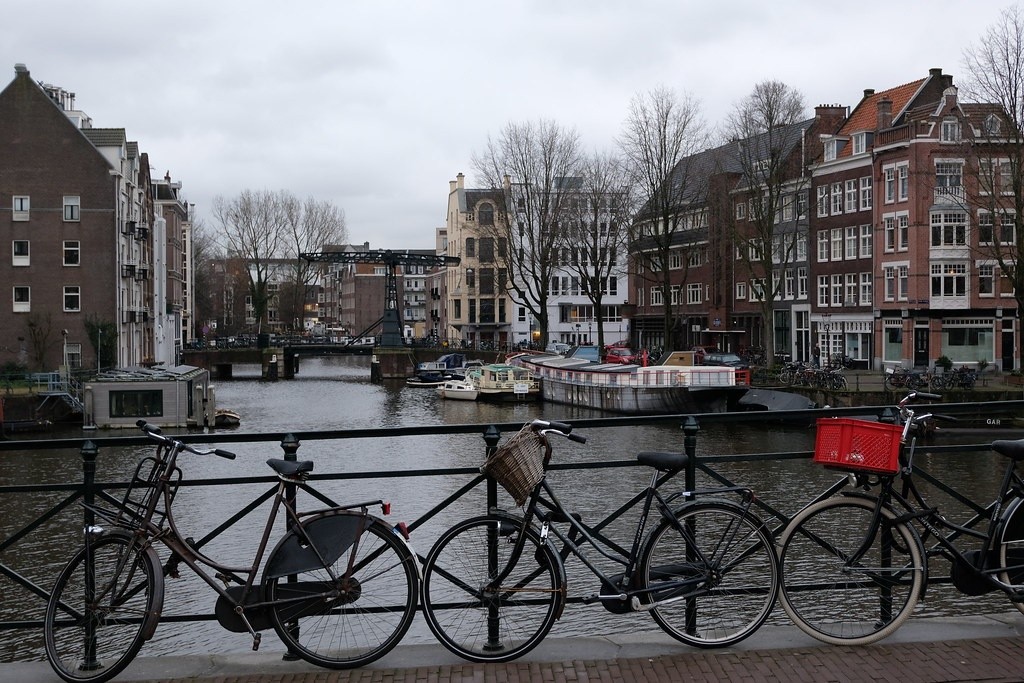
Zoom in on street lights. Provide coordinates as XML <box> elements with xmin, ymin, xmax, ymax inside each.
<box><xmin>528</xmin><ymin>310</ymin><xmax>534</xmax><ymax>350</ymax></box>
<box><xmin>619</xmin><ymin>324</ymin><xmax>622</xmax><ymax>340</ymax></box>
<box><xmin>588</xmin><ymin>322</ymin><xmax>592</xmax><ymax>345</ymax></box>
<box><xmin>158</xmin><ymin>325</ymin><xmax>162</xmax><ymax>362</ymax></box>
<box><xmin>98</xmin><ymin>329</ymin><xmax>102</xmax><ymax>374</ymax></box>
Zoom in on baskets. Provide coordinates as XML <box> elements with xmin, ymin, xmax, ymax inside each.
<box><xmin>813</xmin><ymin>418</ymin><xmax>904</xmax><ymax>473</ymax></box>
<box><xmin>480</xmin><ymin>425</ymin><xmax>544</xmax><ymax>506</ymax></box>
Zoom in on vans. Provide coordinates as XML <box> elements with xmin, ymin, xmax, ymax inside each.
<box><xmin>25</xmin><ymin>373</ymin><xmax>50</xmax><ymax>381</ymax></box>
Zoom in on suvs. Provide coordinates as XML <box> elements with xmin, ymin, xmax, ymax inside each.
<box><xmin>702</xmin><ymin>353</ymin><xmax>749</xmax><ymax>370</ymax></box>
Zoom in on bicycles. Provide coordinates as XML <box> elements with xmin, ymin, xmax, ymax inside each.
<box><xmin>43</xmin><ymin>419</ymin><xmax>424</xmax><ymax>683</ymax></box>
<box><xmin>884</xmin><ymin>364</ymin><xmax>976</xmax><ymax>391</ymax></box>
<box><xmin>776</xmin><ymin>390</ymin><xmax>1024</xmax><ymax>646</ymax></box>
<box><xmin>420</xmin><ymin>417</ymin><xmax>781</xmax><ymax>663</ymax></box>
<box><xmin>780</xmin><ymin>363</ymin><xmax>848</xmax><ymax>396</ymax></box>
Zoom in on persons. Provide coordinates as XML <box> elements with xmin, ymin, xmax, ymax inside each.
<box><xmin>812</xmin><ymin>343</ymin><xmax>820</xmax><ymax>369</ymax></box>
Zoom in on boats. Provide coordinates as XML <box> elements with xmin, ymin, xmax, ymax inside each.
<box><xmin>505</xmin><ymin>352</ymin><xmax>750</xmax><ymax>414</ymax></box>
<box><xmin>405</xmin><ymin>353</ymin><xmax>540</xmax><ymax>402</ymax></box>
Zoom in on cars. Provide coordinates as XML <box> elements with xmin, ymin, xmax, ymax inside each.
<box><xmin>564</xmin><ymin>346</ymin><xmax>599</xmax><ymax>362</ymax></box>
<box><xmin>545</xmin><ymin>343</ymin><xmax>571</xmax><ymax>355</ymax></box>
<box><xmin>690</xmin><ymin>346</ymin><xmax>718</xmax><ymax>364</ymax></box>
<box><xmin>605</xmin><ymin>348</ymin><xmax>639</xmax><ymax>365</ymax></box>
<box><xmin>604</xmin><ymin>339</ymin><xmax>626</xmax><ymax>349</ymax></box>
